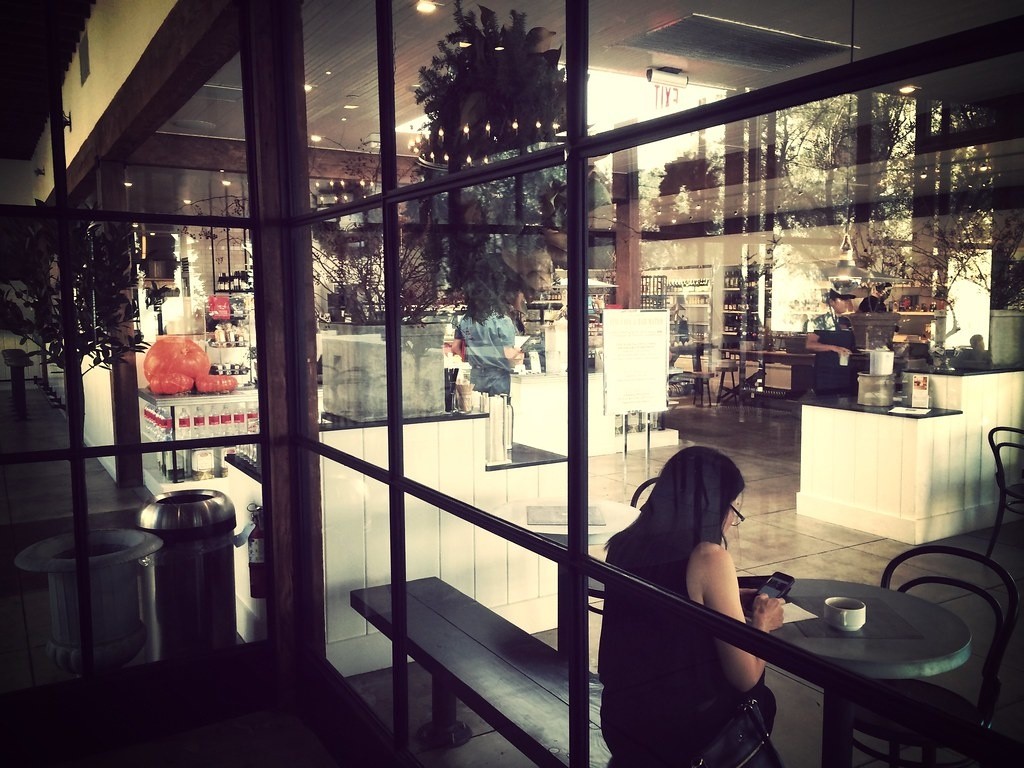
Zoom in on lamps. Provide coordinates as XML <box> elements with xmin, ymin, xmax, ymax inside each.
<box><xmin>920</xmin><ymin>171</ymin><xmax>927</xmax><ymax>178</ymax></box>
<box><xmin>979</xmin><ymin>163</ymin><xmax>987</xmax><ymax>171</ymax></box>
<box><xmin>360</xmin><ymin>180</ymin><xmax>370</xmax><ymax>186</ymax></box>
<box><xmin>780</xmin><ymin>90</ymin><xmax>913</xmax><ymax>295</ymax></box>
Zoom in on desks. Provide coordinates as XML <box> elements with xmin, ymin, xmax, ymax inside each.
<box><xmin>508</xmin><ymin>372</ymin><xmax>616</xmax><ymax>458</ymax></box>
<box><xmin>739</xmin><ymin>579</ymin><xmax>974</xmax><ymax>768</ymax></box>
<box><xmin>491</xmin><ymin>497</ymin><xmax>641</xmax><ymax>592</ymax></box>
<box><xmin>136</xmin><ymin>388</ymin><xmax>325</xmax><ymax>479</ymax></box>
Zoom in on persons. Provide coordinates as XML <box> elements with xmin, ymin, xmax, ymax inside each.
<box><xmin>805</xmin><ymin>289</ymin><xmax>858</xmax><ymax>407</ymax></box>
<box><xmin>452</xmin><ymin>302</ymin><xmax>524</xmax><ymax>402</ymax></box>
<box><xmin>857</xmin><ymin>283</ymin><xmax>892</xmax><ymax>313</ymax></box>
<box><xmin>959</xmin><ymin>334</ymin><xmax>987</xmax><ymax>361</ymax></box>
<box><xmin>597</xmin><ymin>446</ymin><xmax>786</xmax><ymax>768</ymax></box>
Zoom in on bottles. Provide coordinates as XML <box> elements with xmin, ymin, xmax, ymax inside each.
<box><xmin>215</xmin><ymin>323</ymin><xmax>244</xmax><ymax>347</ymax></box>
<box><xmin>641</xmin><ymin>273</ymin><xmax>759</xmax><ymax>360</ymax></box>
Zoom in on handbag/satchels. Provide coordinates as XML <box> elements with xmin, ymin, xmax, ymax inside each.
<box><xmin>460</xmin><ymin>340</ymin><xmax>469</xmax><ymax>363</ymax></box>
<box><xmin>689</xmin><ymin>697</ymin><xmax>784</xmax><ymax>768</ymax></box>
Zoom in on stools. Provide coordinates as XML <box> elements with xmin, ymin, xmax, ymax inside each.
<box><xmin>715</xmin><ymin>367</ymin><xmax>738</xmax><ymax>407</ymax></box>
<box><xmin>692</xmin><ymin>372</ymin><xmax>715</xmax><ymax>407</ymax></box>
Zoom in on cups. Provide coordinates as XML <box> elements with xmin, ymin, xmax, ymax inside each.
<box><xmin>840</xmin><ymin>352</ymin><xmax>848</xmax><ymax>366</ymax></box>
<box><xmin>458</xmin><ymin>392</ymin><xmax>473</xmax><ymax>412</ymax></box>
<box><xmin>824</xmin><ymin>597</ymin><xmax>866</xmax><ymax>632</ymax></box>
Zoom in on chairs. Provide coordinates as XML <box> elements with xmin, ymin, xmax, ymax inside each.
<box><xmin>985</xmin><ymin>427</ymin><xmax>1024</xmax><ymax>558</ymax></box>
<box><xmin>852</xmin><ymin>545</ymin><xmax>1020</xmax><ymax>768</ymax></box>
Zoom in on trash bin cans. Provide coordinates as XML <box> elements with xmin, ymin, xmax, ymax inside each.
<box><xmin>15</xmin><ymin>528</ymin><xmax>164</xmax><ymax>675</ymax></box>
<box><xmin>134</xmin><ymin>488</ymin><xmax>238</xmax><ymax>660</ymax></box>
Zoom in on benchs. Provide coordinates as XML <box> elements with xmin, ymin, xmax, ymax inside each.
<box><xmin>350</xmin><ymin>576</ymin><xmax>613</xmax><ymax>768</ymax></box>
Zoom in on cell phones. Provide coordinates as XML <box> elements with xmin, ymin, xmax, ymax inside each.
<box><xmin>745</xmin><ymin>571</ymin><xmax>795</xmax><ymax>612</ymax></box>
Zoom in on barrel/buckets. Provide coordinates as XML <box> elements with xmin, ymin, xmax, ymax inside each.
<box><xmin>858</xmin><ymin>373</ymin><xmax>897</xmax><ymax>407</ymax></box>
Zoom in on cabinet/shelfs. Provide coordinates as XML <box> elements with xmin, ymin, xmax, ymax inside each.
<box><xmin>316</xmin><ymin>191</ymin><xmax>341</xmax><ymax>223</ymax></box>
<box><xmin>836</xmin><ymin>252</ymin><xmax>956</xmax><ymax>357</ymax></box>
<box><xmin>525</xmin><ymin>268</ymin><xmax>616</xmax><ymax>309</ymax></box>
<box><xmin>641</xmin><ymin>278</ymin><xmax>711</xmax><ymax>375</ymax></box>
<box><xmin>712</xmin><ymin>262</ymin><xmax>772</xmax><ymax>381</ymax></box>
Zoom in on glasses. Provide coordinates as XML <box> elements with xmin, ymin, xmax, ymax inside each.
<box><xmin>729</xmin><ymin>503</ymin><xmax>745</xmax><ymax>527</ymax></box>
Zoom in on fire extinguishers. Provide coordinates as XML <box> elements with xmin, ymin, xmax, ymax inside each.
<box><xmin>247</xmin><ymin>503</ymin><xmax>268</xmax><ymax>598</ymax></box>
